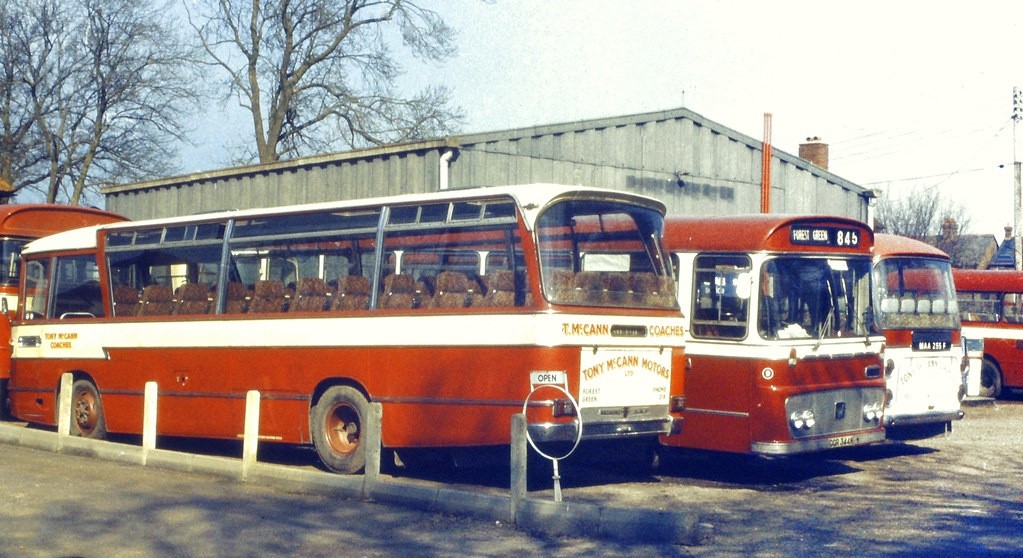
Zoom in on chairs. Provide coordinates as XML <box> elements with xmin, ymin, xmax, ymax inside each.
<box><xmin>605</xmin><ymin>270</ymin><xmax>633</xmax><ymax>305</ymax></box>
<box><xmin>915</xmin><ymin>300</ymin><xmax>931</xmax><ymax>327</ymax></box>
<box><xmin>378</xmin><ymin>274</ymin><xmax>416</xmax><ymax>308</ymax></box>
<box><xmin>576</xmin><ymin>271</ymin><xmax>604</xmax><ymax>304</ymax></box>
<box><xmin>633</xmin><ymin>272</ymin><xmax>656</xmax><ymax>304</ymax></box>
<box><xmin>881</xmin><ymin>299</ymin><xmax>901</xmax><ymax>328</ymax></box>
<box><xmin>526</xmin><ymin>268</ymin><xmax>576</xmax><ymax>308</ymax></box>
<box><xmin>286</xmin><ymin>277</ymin><xmax>326</xmax><ymax>312</ymax></box>
<box><xmin>944</xmin><ymin>300</ymin><xmax>958</xmax><ymax>327</ymax></box>
<box><xmin>328</xmin><ymin>276</ymin><xmax>368</xmax><ymax>311</ymax></box>
<box><xmin>247</xmin><ymin>281</ymin><xmax>284</xmax><ymax>313</ymax></box>
<box><xmin>208</xmin><ymin>282</ymin><xmax>248</xmax><ymax>314</ymax></box>
<box><xmin>114</xmin><ymin>286</ymin><xmax>140</xmax><ymax>316</ymax></box>
<box><xmin>415</xmin><ymin>276</ymin><xmax>435</xmax><ymax>306</ymax></box>
<box><xmin>479</xmin><ymin>270</ymin><xmax>523</xmax><ymax>307</ymax></box>
<box><xmin>901</xmin><ymin>299</ymin><xmax>916</xmax><ymax>327</ymax></box>
<box><xmin>172</xmin><ymin>283</ymin><xmax>209</xmax><ymax>314</ymax></box>
<box><xmin>138</xmin><ymin>286</ymin><xmax>173</xmax><ymax>315</ymax></box>
<box><xmin>427</xmin><ymin>271</ymin><xmax>468</xmax><ymax>309</ymax></box>
<box><xmin>930</xmin><ymin>300</ymin><xmax>946</xmax><ymax>327</ymax></box>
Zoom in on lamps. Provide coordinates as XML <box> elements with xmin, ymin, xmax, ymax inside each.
<box><xmin>676</xmin><ymin>168</ymin><xmax>691</xmax><ymax>176</ymax></box>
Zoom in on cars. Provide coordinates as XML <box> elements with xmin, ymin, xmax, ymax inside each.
<box><xmin>869</xmin><ymin>231</ymin><xmax>968</xmax><ymax>432</ymax></box>
<box><xmin>884</xmin><ymin>267</ymin><xmax>1023</xmax><ymax>398</ymax></box>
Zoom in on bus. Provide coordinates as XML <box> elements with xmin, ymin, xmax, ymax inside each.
<box><xmin>0</xmin><ymin>184</ymin><xmax>694</xmax><ymax>479</ymax></box>
<box><xmin>252</xmin><ymin>210</ymin><xmax>895</xmax><ymax>468</ymax></box>
<box><xmin>0</xmin><ymin>203</ymin><xmax>139</xmax><ymax>384</ymax></box>
